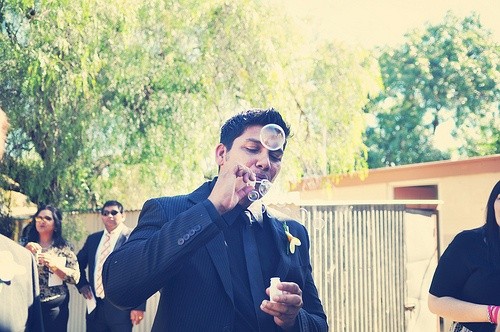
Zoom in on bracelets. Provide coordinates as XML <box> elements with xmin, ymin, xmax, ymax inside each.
<box><xmin>487</xmin><ymin>304</ymin><xmax>500</xmax><ymax>325</ymax></box>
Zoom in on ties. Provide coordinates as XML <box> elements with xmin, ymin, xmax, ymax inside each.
<box><xmin>96</xmin><ymin>231</ymin><xmax>111</xmax><ymax>299</ymax></box>
<box><xmin>239</xmin><ymin>210</ymin><xmax>277</xmax><ymax>331</ymax></box>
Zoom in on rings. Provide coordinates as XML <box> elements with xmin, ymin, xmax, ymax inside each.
<box><xmin>139</xmin><ymin>316</ymin><xmax>143</xmax><ymax>320</ymax></box>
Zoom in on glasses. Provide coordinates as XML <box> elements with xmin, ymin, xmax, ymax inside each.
<box><xmin>35</xmin><ymin>215</ymin><xmax>53</xmax><ymax>222</ymax></box>
<box><xmin>100</xmin><ymin>209</ymin><xmax>121</xmax><ymax>216</ymax></box>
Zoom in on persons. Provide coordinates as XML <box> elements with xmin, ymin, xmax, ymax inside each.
<box><xmin>429</xmin><ymin>180</ymin><xmax>500</xmax><ymax>332</ymax></box>
<box><xmin>21</xmin><ymin>204</ymin><xmax>81</xmax><ymax>332</ymax></box>
<box><xmin>102</xmin><ymin>107</ymin><xmax>329</xmax><ymax>332</ymax></box>
<box><xmin>74</xmin><ymin>200</ymin><xmax>146</xmax><ymax>332</ymax></box>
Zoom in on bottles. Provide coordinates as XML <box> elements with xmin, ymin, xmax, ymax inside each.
<box><xmin>37</xmin><ymin>249</ymin><xmax>44</xmax><ymax>266</ymax></box>
<box><xmin>270</xmin><ymin>278</ymin><xmax>282</xmax><ymax>303</ymax></box>
